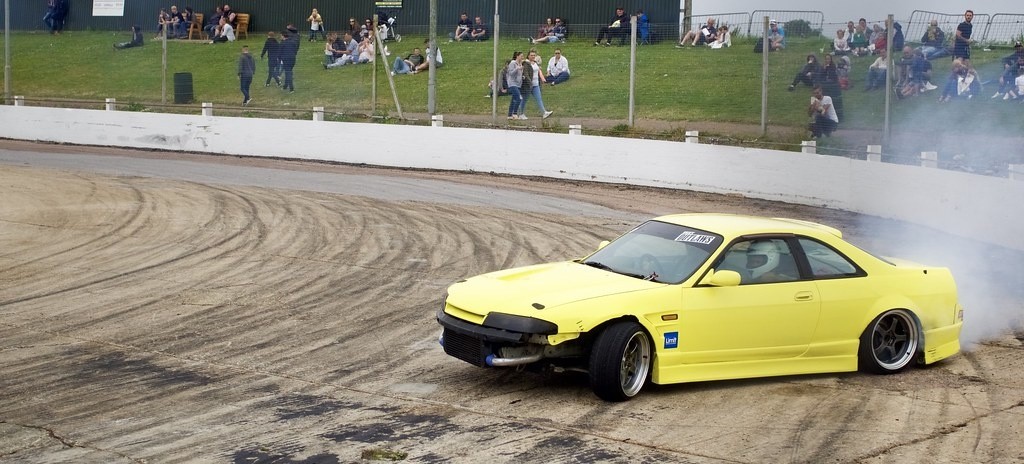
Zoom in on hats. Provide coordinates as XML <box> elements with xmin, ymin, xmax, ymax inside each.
<box><xmin>1015</xmin><ymin>42</ymin><xmax>1023</xmax><ymax>47</ymax></box>
<box><xmin>424</xmin><ymin>39</ymin><xmax>429</xmax><ymax>43</ymax></box>
<box><xmin>770</xmin><ymin>20</ymin><xmax>777</xmax><ymax>24</ymax></box>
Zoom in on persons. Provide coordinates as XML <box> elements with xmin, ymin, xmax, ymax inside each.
<box><xmin>703</xmin><ymin>26</ymin><xmax>731</xmax><ymax>49</ymax></box>
<box><xmin>466</xmin><ymin>16</ymin><xmax>490</xmax><ymax>42</ymax></box>
<box><xmin>762</xmin><ymin>20</ymin><xmax>786</xmax><ymax>52</ymax></box>
<box><xmin>546</xmin><ymin>48</ymin><xmax>571</xmax><ymax>86</ymax></box>
<box><xmin>308</xmin><ymin>8</ymin><xmax>374</xmax><ymax>69</ymax></box>
<box><xmin>237</xmin><ymin>45</ymin><xmax>256</xmax><ymax>106</ymax></box>
<box><xmin>745</xmin><ymin>239</ymin><xmax>784</xmax><ymax>283</ymax></box>
<box><xmin>593</xmin><ymin>7</ymin><xmax>630</xmax><ymax>46</ymax></box>
<box><xmin>154</xmin><ymin>4</ymin><xmax>238</xmax><ymax>44</ymax></box>
<box><xmin>449</xmin><ymin>12</ymin><xmax>472</xmax><ymax>41</ymax></box>
<box><xmin>260</xmin><ymin>23</ymin><xmax>300</xmax><ymax>94</ymax></box>
<box><xmin>113</xmin><ymin>25</ymin><xmax>144</xmax><ymax>50</ymax></box>
<box><xmin>674</xmin><ymin>18</ymin><xmax>717</xmax><ymax>48</ymax></box>
<box><xmin>391</xmin><ymin>47</ymin><xmax>424</xmax><ymax>76</ymax></box>
<box><xmin>409</xmin><ymin>38</ymin><xmax>442</xmax><ymax>73</ymax></box>
<box><xmin>788</xmin><ymin>10</ymin><xmax>1024</xmax><ymax>140</ymax></box>
<box><xmin>528</xmin><ymin>18</ymin><xmax>567</xmax><ymax>45</ymax></box>
<box><xmin>488</xmin><ymin>48</ymin><xmax>553</xmax><ymax>120</ymax></box>
<box><xmin>617</xmin><ymin>10</ymin><xmax>650</xmax><ymax>47</ymax></box>
<box><xmin>43</xmin><ymin>0</ymin><xmax>70</xmax><ymax>34</ymax></box>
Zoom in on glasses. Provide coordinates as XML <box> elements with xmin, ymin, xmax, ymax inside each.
<box><xmin>350</xmin><ymin>21</ymin><xmax>355</xmax><ymax>23</ymax></box>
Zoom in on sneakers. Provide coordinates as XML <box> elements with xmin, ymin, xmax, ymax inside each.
<box><xmin>508</xmin><ymin>117</ymin><xmax>513</xmax><ymax>119</ymax></box>
<box><xmin>519</xmin><ymin>114</ymin><xmax>528</xmax><ymax>119</ymax></box>
<box><xmin>513</xmin><ymin>115</ymin><xmax>520</xmax><ymax>120</ymax></box>
<box><xmin>543</xmin><ymin>111</ymin><xmax>553</xmax><ymax>119</ymax></box>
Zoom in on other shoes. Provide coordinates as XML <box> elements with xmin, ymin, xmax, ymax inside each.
<box><xmin>1003</xmin><ymin>94</ymin><xmax>1009</xmax><ymax>99</ymax></box>
<box><xmin>391</xmin><ymin>72</ymin><xmax>396</xmax><ymax>76</ymax></box>
<box><xmin>675</xmin><ymin>44</ymin><xmax>684</xmax><ymax>48</ymax></box>
<box><xmin>321</xmin><ymin>62</ymin><xmax>327</xmax><ymax>69</ymax></box>
<box><xmin>991</xmin><ymin>91</ymin><xmax>1003</xmax><ymax>98</ymax></box>
<box><xmin>529</xmin><ymin>36</ymin><xmax>534</xmax><ymax>44</ymax></box>
<box><xmin>242</xmin><ymin>82</ymin><xmax>295</xmax><ymax>106</ymax></box>
<box><xmin>789</xmin><ymin>87</ymin><xmax>793</xmax><ymax>90</ymax></box>
<box><xmin>895</xmin><ymin>89</ymin><xmax>903</xmax><ymax>100</ymax></box>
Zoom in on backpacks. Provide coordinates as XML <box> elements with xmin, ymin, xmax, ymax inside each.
<box><xmin>754</xmin><ymin>37</ymin><xmax>772</xmax><ymax>52</ymax></box>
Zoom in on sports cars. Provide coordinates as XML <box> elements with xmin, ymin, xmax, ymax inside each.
<box><xmin>435</xmin><ymin>211</ymin><xmax>962</xmax><ymax>400</ymax></box>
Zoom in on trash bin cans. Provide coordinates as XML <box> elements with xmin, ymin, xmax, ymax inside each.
<box><xmin>174</xmin><ymin>72</ymin><xmax>194</xmax><ymax>104</ymax></box>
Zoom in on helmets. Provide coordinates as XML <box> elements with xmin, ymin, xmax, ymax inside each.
<box><xmin>744</xmin><ymin>239</ymin><xmax>780</xmax><ymax>279</ymax></box>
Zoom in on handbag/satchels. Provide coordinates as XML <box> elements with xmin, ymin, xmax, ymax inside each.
<box><xmin>925</xmin><ymin>81</ymin><xmax>938</xmax><ymax>90</ymax></box>
<box><xmin>839</xmin><ymin>76</ymin><xmax>848</xmax><ymax>88</ymax></box>
<box><xmin>711</xmin><ymin>42</ymin><xmax>722</xmax><ymax>49</ymax></box>
<box><xmin>612</xmin><ymin>20</ymin><xmax>620</xmax><ymax>27</ymax></box>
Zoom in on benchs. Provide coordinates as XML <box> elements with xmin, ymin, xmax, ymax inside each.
<box><xmin>231</xmin><ymin>12</ymin><xmax>249</xmax><ymax>40</ymax></box>
<box><xmin>180</xmin><ymin>12</ymin><xmax>204</xmax><ymax>39</ymax></box>
